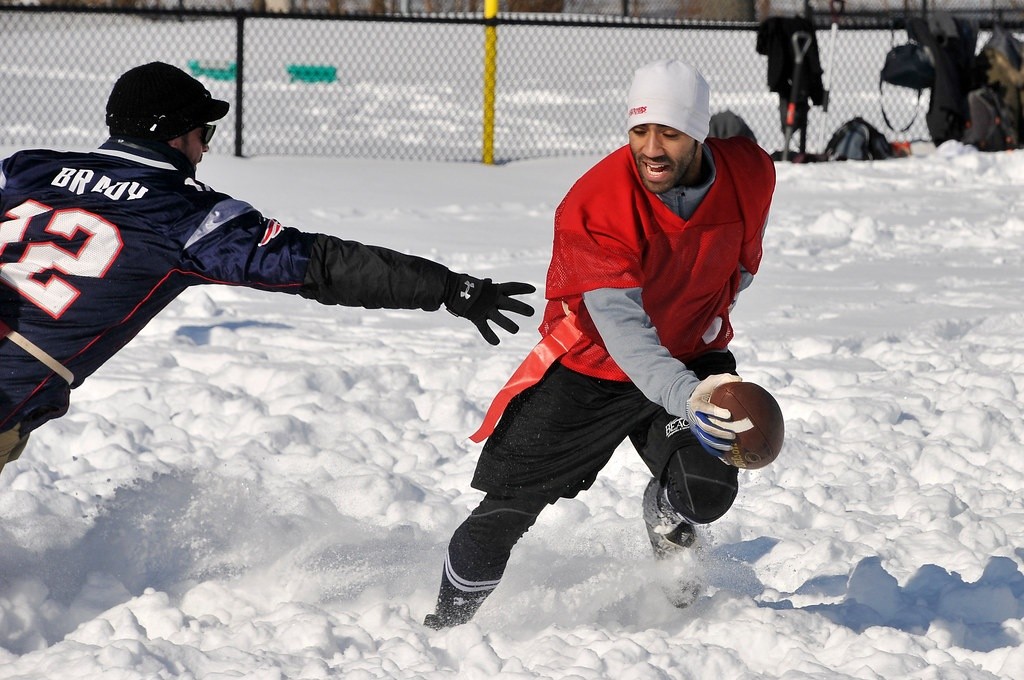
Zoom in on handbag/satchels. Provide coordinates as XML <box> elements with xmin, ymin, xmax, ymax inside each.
<box><xmin>881</xmin><ymin>43</ymin><xmax>936</xmax><ymax>89</ymax></box>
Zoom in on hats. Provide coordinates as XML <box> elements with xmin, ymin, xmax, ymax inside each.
<box><xmin>626</xmin><ymin>58</ymin><xmax>710</xmax><ymax>143</ymax></box>
<box><xmin>105</xmin><ymin>62</ymin><xmax>231</xmax><ymax>143</ymax></box>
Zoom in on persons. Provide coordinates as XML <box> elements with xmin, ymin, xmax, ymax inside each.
<box><xmin>1</xmin><ymin>63</ymin><xmax>538</xmax><ymax>474</ymax></box>
<box><xmin>424</xmin><ymin>57</ymin><xmax>778</xmax><ymax>632</ymax></box>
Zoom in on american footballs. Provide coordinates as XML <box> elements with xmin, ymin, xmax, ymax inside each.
<box><xmin>707</xmin><ymin>381</ymin><xmax>785</xmax><ymax>469</ymax></box>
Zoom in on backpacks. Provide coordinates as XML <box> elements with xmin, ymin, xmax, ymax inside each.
<box><xmin>824</xmin><ymin>116</ymin><xmax>893</xmax><ymax>161</ymax></box>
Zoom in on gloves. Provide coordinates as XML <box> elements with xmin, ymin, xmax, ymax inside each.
<box><xmin>686</xmin><ymin>372</ymin><xmax>742</xmax><ymax>457</ymax></box>
<box><xmin>445</xmin><ymin>268</ymin><xmax>536</xmax><ymax>346</ymax></box>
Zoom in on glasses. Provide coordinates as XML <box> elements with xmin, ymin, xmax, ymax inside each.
<box><xmin>197</xmin><ymin>120</ymin><xmax>217</xmax><ymax>145</ymax></box>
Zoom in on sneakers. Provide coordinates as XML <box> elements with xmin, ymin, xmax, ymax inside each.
<box><xmin>643</xmin><ymin>477</ymin><xmax>700</xmax><ymax>609</ymax></box>
